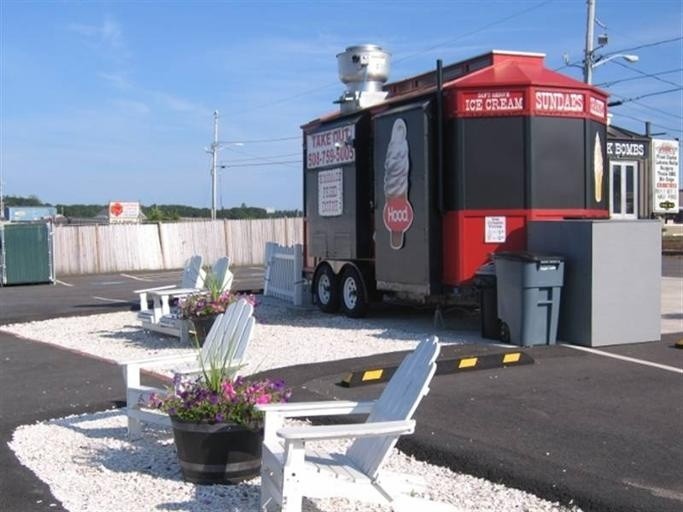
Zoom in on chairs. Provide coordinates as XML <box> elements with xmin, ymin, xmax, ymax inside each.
<box><xmin>116</xmin><ymin>299</ymin><xmax>255</xmax><ymax>442</ymax></box>
<box><xmin>254</xmin><ymin>335</ymin><xmax>458</xmax><ymax>512</ymax></box>
<box><xmin>134</xmin><ymin>255</ymin><xmax>233</xmax><ymax>343</ymax></box>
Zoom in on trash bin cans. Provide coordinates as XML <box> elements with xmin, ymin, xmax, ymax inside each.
<box><xmin>474</xmin><ymin>260</ymin><xmax>500</xmax><ymax>341</ymax></box>
<box><xmin>491</xmin><ymin>250</ymin><xmax>566</xmax><ymax>348</ymax></box>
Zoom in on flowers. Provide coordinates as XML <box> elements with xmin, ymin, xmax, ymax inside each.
<box><xmin>137</xmin><ymin>330</ymin><xmax>294</xmax><ymax>425</ymax></box>
<box><xmin>176</xmin><ymin>269</ymin><xmax>262</xmax><ymax>319</ymax></box>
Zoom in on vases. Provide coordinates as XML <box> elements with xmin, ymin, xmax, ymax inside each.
<box><xmin>169</xmin><ymin>414</ymin><xmax>264</xmax><ymax>484</ymax></box>
<box><xmin>192</xmin><ymin>314</ymin><xmax>222</xmax><ymax>347</ymax></box>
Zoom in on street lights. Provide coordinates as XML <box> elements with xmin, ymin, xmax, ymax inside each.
<box><xmin>212</xmin><ymin>142</ymin><xmax>244</xmax><ymax>219</ymax></box>
<box><xmin>583</xmin><ymin>52</ymin><xmax>640</xmax><ymax>86</ymax></box>
